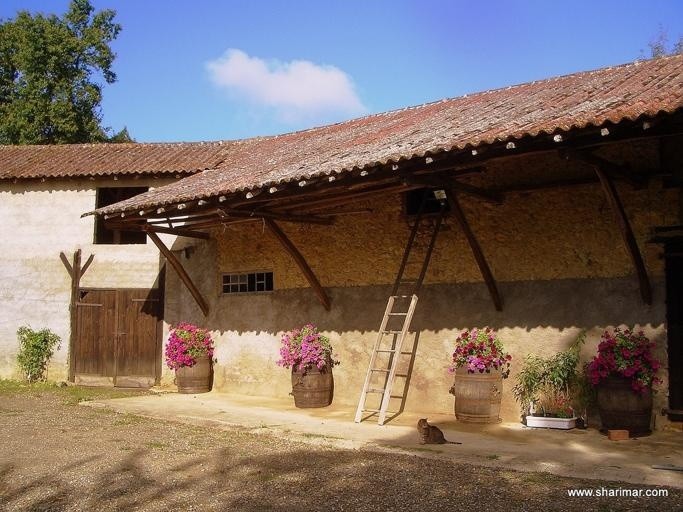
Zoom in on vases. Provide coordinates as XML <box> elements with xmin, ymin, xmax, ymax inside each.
<box><xmin>175</xmin><ymin>356</ymin><xmax>213</xmax><ymax>394</ymax></box>
<box><xmin>596</xmin><ymin>379</ymin><xmax>652</xmax><ymax>438</ymax></box>
<box><xmin>291</xmin><ymin>364</ymin><xmax>333</xmax><ymax>409</ymax></box>
<box><xmin>453</xmin><ymin>374</ymin><xmax>503</xmax><ymax>424</ymax></box>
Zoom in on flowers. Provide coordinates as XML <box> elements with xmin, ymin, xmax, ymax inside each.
<box><xmin>165</xmin><ymin>320</ymin><xmax>215</xmax><ymax>371</ymax></box>
<box><xmin>585</xmin><ymin>324</ymin><xmax>666</xmax><ymax>396</ymax></box>
<box><xmin>274</xmin><ymin>321</ymin><xmax>341</xmax><ymax>372</ymax></box>
<box><xmin>447</xmin><ymin>325</ymin><xmax>512</xmax><ymax>378</ymax></box>
<box><xmin>555</xmin><ymin>397</ymin><xmax>577</xmax><ymax>418</ymax></box>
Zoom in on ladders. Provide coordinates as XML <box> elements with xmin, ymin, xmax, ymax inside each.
<box><xmin>354</xmin><ymin>189</ymin><xmax>447</xmax><ymax>426</ymax></box>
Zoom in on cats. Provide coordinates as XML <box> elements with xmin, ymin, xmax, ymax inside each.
<box><xmin>416</xmin><ymin>418</ymin><xmax>462</xmax><ymax>444</ymax></box>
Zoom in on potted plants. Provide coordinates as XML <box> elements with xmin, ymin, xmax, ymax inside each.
<box><xmin>512</xmin><ymin>330</ymin><xmax>585</xmax><ymax>430</ymax></box>
